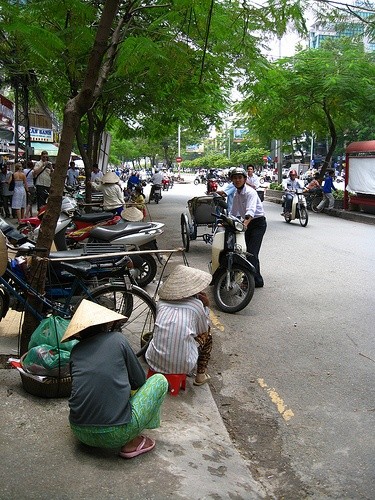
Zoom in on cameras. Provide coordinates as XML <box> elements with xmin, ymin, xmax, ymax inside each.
<box><xmin>47</xmin><ymin>161</ymin><xmax>52</xmax><ymax>168</ymax></box>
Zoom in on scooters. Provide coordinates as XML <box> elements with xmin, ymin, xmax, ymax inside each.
<box><xmin>0</xmin><ymin>166</ymin><xmax>174</xmax><ymax>332</ymax></box>
<box><xmin>194</xmin><ymin>172</ymin><xmax>231</xmax><ymax>195</ymax></box>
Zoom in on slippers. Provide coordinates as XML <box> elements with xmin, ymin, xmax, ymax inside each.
<box><xmin>194</xmin><ymin>372</ymin><xmax>211</xmax><ymax>385</ymax></box>
<box><xmin>120</xmin><ymin>435</ymin><xmax>155</xmax><ymax>458</ymax></box>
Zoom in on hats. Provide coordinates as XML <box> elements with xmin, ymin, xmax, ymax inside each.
<box><xmin>101</xmin><ymin>171</ymin><xmax>119</xmax><ymax>183</ymax></box>
<box><xmin>158</xmin><ymin>265</ymin><xmax>212</xmax><ymax>300</ymax></box>
<box><xmin>60</xmin><ymin>299</ymin><xmax>128</xmax><ymax>342</ymax></box>
<box><xmin>121</xmin><ymin>207</ymin><xmax>144</xmax><ymax>222</ymax></box>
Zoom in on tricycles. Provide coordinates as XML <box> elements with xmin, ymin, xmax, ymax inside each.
<box><xmin>181</xmin><ymin>192</ymin><xmax>233</xmax><ymax>253</ymax></box>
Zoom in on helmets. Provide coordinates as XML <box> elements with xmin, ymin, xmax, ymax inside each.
<box><xmin>228</xmin><ymin>167</ymin><xmax>236</xmax><ymax>176</ymax></box>
<box><xmin>231</xmin><ymin>167</ymin><xmax>247</xmax><ymax>180</ymax></box>
<box><xmin>132</xmin><ymin>172</ymin><xmax>135</xmax><ymax>176</ymax></box>
<box><xmin>290</xmin><ymin>170</ymin><xmax>297</xmax><ymax>175</ymax></box>
<box><xmin>155</xmin><ymin>169</ymin><xmax>159</xmax><ymax>171</ymax></box>
<box><xmin>210</xmin><ymin>168</ymin><xmax>215</xmax><ymax>171</ymax></box>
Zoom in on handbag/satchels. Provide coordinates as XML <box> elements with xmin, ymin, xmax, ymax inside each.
<box><xmin>33</xmin><ymin>177</ymin><xmax>37</xmax><ymax>185</ymax></box>
<box><xmin>28</xmin><ymin>315</ymin><xmax>80</xmax><ymax>352</ymax></box>
<box><xmin>9</xmin><ymin>174</ymin><xmax>15</xmax><ymax>191</ymax></box>
<box><xmin>24</xmin><ymin>344</ymin><xmax>71</xmax><ymax>379</ymax></box>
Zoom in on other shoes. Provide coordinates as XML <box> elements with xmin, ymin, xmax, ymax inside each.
<box><xmin>315</xmin><ymin>208</ymin><xmax>320</xmax><ymax>213</ymax></box>
<box><xmin>328</xmin><ymin>209</ymin><xmax>334</xmax><ymax>212</ymax></box>
<box><xmin>285</xmin><ymin>212</ymin><xmax>290</xmax><ymax>218</ymax></box>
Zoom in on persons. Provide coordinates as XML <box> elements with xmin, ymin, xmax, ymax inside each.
<box><xmin>316</xmin><ymin>170</ymin><xmax>339</xmax><ymax>213</ymax></box>
<box><xmin>69</xmin><ymin>306</ymin><xmax>169</xmax><ymax>459</ymax></box>
<box><xmin>302</xmin><ymin>172</ymin><xmax>324</xmax><ymax>198</ymax></box>
<box><xmin>281</xmin><ymin>170</ymin><xmax>309</xmax><ymax>219</ymax></box>
<box><xmin>91</xmin><ymin>164</ymin><xmax>104</xmax><ymax>185</ymax></box>
<box><xmin>146</xmin><ymin>168</ymin><xmax>165</xmax><ymax>204</ymax></box>
<box><xmin>245</xmin><ymin>165</ymin><xmax>260</xmax><ymax>190</ymax></box>
<box><xmin>0</xmin><ymin>156</ymin><xmax>37</xmax><ymax>219</ymax></box>
<box><xmin>207</xmin><ymin>168</ymin><xmax>216</xmax><ymax>193</ymax></box>
<box><xmin>229</xmin><ymin>168</ymin><xmax>266</xmax><ymax>287</ymax></box>
<box><xmin>8</xmin><ymin>162</ymin><xmax>31</xmax><ymax>224</ymax></box>
<box><xmin>32</xmin><ymin>150</ymin><xmax>54</xmax><ymax>214</ymax></box>
<box><xmin>65</xmin><ymin>161</ymin><xmax>79</xmax><ymax>187</ymax></box>
<box><xmin>106</xmin><ymin>167</ymin><xmax>171</xmax><ymax>191</ymax></box>
<box><xmin>198</xmin><ymin>166</ymin><xmax>236</xmax><ymax>216</ymax></box>
<box><xmin>96</xmin><ymin>174</ymin><xmax>125</xmax><ymax>217</ymax></box>
<box><xmin>145</xmin><ymin>267</ymin><xmax>213</xmax><ymax>386</ymax></box>
<box><xmin>126</xmin><ymin>185</ymin><xmax>145</xmax><ymax>217</ymax></box>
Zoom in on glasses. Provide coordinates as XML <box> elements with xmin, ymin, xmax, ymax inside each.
<box><xmin>42</xmin><ymin>155</ymin><xmax>48</xmax><ymax>158</ymax></box>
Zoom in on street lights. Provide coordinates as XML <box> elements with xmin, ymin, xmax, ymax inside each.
<box><xmin>178</xmin><ymin>124</ymin><xmax>188</xmax><ymax>176</ymax></box>
<box><xmin>308</xmin><ymin>136</ymin><xmax>313</xmax><ymax>171</ymax></box>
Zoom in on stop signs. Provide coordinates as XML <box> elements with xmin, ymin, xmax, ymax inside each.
<box><xmin>176</xmin><ymin>157</ymin><xmax>182</xmax><ymax>163</ymax></box>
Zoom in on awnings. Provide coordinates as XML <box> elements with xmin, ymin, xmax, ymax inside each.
<box><xmin>70</xmin><ymin>152</ymin><xmax>83</xmax><ymax>160</ymax></box>
<box><xmin>18</xmin><ymin>141</ymin><xmax>77</xmax><ymax>157</ymax></box>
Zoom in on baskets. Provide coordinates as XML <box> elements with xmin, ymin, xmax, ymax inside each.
<box><xmin>19</xmin><ymin>353</ymin><xmax>72</xmax><ymax>398</ymax></box>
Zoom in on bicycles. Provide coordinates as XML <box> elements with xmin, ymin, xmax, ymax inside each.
<box><xmin>0</xmin><ymin>234</ymin><xmax>159</xmax><ymax>358</ymax></box>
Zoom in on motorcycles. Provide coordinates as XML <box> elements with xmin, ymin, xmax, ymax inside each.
<box><xmin>280</xmin><ymin>183</ymin><xmax>310</xmax><ymax>227</ymax></box>
<box><xmin>207</xmin><ymin>200</ymin><xmax>258</xmax><ymax>314</ymax></box>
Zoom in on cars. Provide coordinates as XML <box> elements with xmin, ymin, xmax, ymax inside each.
<box><xmin>322</xmin><ymin>175</ymin><xmax>346</xmax><ymax>191</ymax></box>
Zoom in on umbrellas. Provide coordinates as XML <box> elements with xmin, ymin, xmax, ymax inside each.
<box><xmin>68</xmin><ymin>159</ymin><xmax>86</xmax><ymax>169</ymax></box>
